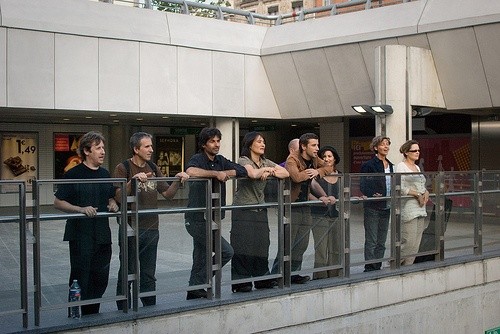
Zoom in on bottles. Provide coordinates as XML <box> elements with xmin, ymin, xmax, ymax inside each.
<box><xmin>69</xmin><ymin>279</ymin><xmax>81</xmax><ymax>320</ymax></box>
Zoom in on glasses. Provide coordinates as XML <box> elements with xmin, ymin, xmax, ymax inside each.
<box><xmin>409</xmin><ymin>150</ymin><xmax>420</xmax><ymax>153</ymax></box>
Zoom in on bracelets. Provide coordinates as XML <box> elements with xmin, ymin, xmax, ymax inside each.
<box><xmin>416</xmin><ymin>192</ymin><xmax>420</xmax><ymax>199</ymax></box>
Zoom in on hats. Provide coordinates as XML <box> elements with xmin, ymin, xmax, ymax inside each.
<box><xmin>318</xmin><ymin>145</ymin><xmax>340</xmax><ymax>164</ymax></box>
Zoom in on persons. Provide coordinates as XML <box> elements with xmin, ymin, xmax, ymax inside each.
<box><xmin>156</xmin><ymin>150</ymin><xmax>182</xmax><ymax>177</ymax></box>
<box><xmin>359</xmin><ymin>134</ymin><xmax>395</xmax><ymax>273</ymax></box>
<box><xmin>231</xmin><ymin>130</ymin><xmax>279</xmax><ymax>292</ymax></box>
<box><xmin>383</xmin><ymin>139</ymin><xmax>429</xmax><ymax>268</ymax></box>
<box><xmin>309</xmin><ymin>147</ymin><xmax>344</xmax><ymax>280</ymax></box>
<box><xmin>111</xmin><ymin>132</ymin><xmax>189</xmax><ymax>310</ymax></box>
<box><xmin>184</xmin><ymin>127</ymin><xmax>248</xmax><ymax>300</ymax></box>
<box><xmin>271</xmin><ymin>133</ymin><xmax>336</xmax><ymax>286</ymax></box>
<box><xmin>54</xmin><ymin>130</ymin><xmax>119</xmax><ymax>318</ymax></box>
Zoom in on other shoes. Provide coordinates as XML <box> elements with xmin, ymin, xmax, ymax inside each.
<box><xmin>186</xmin><ymin>287</ymin><xmax>214</xmax><ymax>300</ymax></box>
<box><xmin>291</xmin><ymin>275</ymin><xmax>310</xmax><ymax>284</ymax></box>
